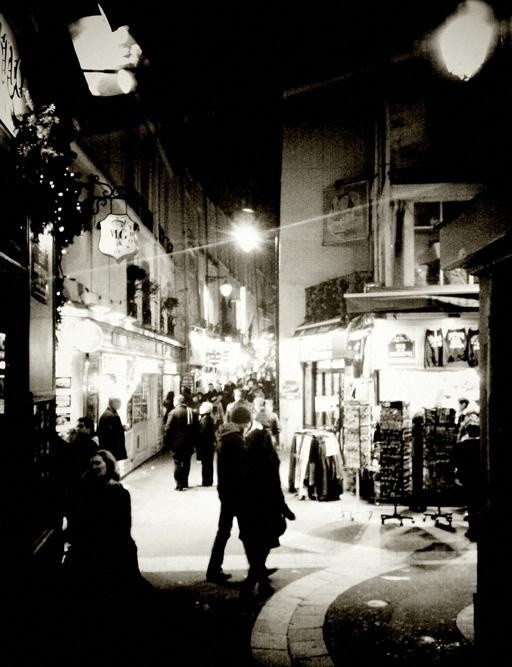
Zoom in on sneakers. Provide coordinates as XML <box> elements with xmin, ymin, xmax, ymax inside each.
<box><xmin>205</xmin><ymin>566</ymin><xmax>280</xmax><ymax>594</ymax></box>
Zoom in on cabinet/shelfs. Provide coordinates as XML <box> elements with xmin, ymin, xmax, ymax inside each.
<box><xmin>345</xmin><ymin>401</ymin><xmax>381</xmax><ymax>500</ymax></box>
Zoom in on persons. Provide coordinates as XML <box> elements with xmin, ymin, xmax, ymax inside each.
<box><xmin>159</xmin><ymin>369</ymin><xmax>295</xmax><ymax>601</ymax></box>
<box><xmin>455</xmin><ymin>396</ymin><xmax>469</xmax><ymax>433</ymax></box>
<box><xmin>57</xmin><ymin>397</ymin><xmax>153</xmax><ymax>667</ymax></box>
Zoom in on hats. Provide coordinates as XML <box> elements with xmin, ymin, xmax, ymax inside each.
<box><xmin>230</xmin><ymin>406</ymin><xmax>253</xmax><ymax>425</ymax></box>
